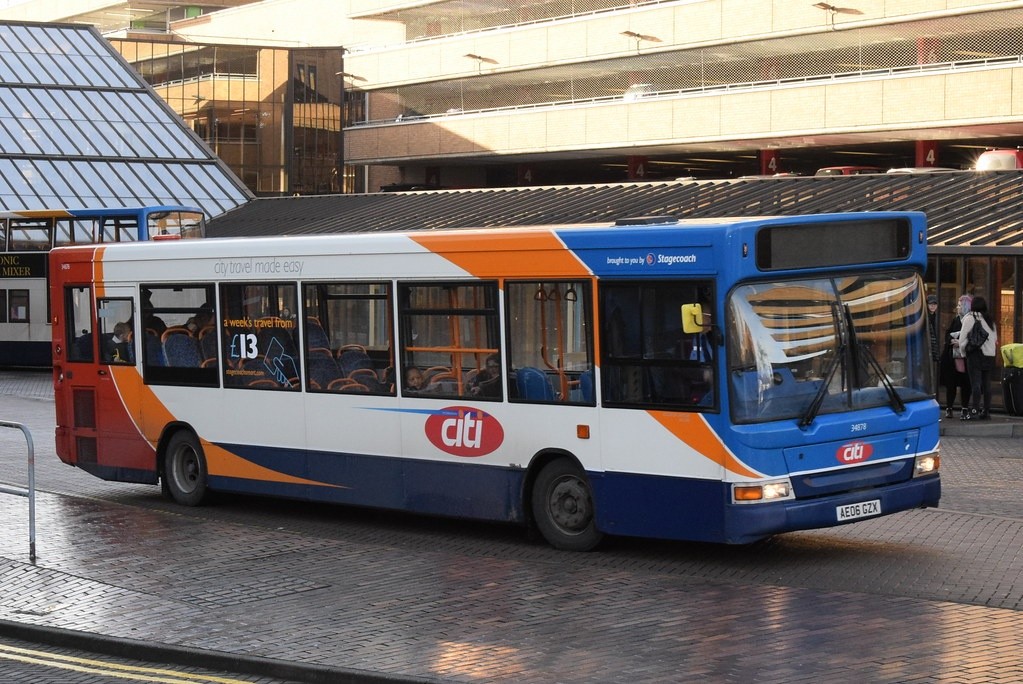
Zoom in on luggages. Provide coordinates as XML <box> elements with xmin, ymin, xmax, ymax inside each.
<box><xmin>1001</xmin><ymin>367</ymin><xmax>1023</xmax><ymax>416</ymax></box>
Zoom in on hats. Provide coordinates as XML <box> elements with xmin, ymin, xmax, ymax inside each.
<box><xmin>926</xmin><ymin>295</ymin><xmax>937</xmax><ymax>304</ymax></box>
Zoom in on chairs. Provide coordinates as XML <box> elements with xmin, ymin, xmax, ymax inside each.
<box><xmin>76</xmin><ymin>316</ymin><xmax>595</xmax><ymax>404</ymax></box>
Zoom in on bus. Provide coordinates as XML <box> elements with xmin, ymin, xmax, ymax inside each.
<box><xmin>0</xmin><ymin>205</ymin><xmax>209</xmax><ymax>367</ymax></box>
<box><xmin>47</xmin><ymin>214</ymin><xmax>947</xmax><ymax>554</ymax></box>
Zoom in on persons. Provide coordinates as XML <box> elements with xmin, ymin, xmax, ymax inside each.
<box><xmin>925</xmin><ymin>295</ymin><xmax>947</xmax><ymax>408</ymax></box>
<box><xmin>281</xmin><ymin>307</ymin><xmax>293</xmax><ymax>319</ymax></box>
<box><xmin>404</xmin><ymin>365</ymin><xmax>424</xmax><ymax>391</ymax></box>
<box><xmin>104</xmin><ymin>300</ymin><xmax>216</xmax><ymax>363</ymax></box>
<box><xmin>467</xmin><ymin>355</ymin><xmax>501</xmax><ymax>396</ymax></box>
<box><xmin>945</xmin><ymin>296</ymin><xmax>997</xmax><ymax>421</ymax></box>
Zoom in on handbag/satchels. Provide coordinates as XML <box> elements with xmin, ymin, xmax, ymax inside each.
<box><xmin>965</xmin><ymin>311</ymin><xmax>989</xmax><ymax>355</ymax></box>
<box><xmin>1000</xmin><ymin>343</ymin><xmax>1023</xmax><ymax>368</ymax></box>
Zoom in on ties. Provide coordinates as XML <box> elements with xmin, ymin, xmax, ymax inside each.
<box><xmin>701</xmin><ymin>334</ymin><xmax>712</xmax><ymax>364</ymax></box>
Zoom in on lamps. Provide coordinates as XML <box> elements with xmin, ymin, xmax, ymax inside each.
<box><xmin>621</xmin><ymin>31</ymin><xmax>640</xmax><ymax>38</ymax></box>
<box><xmin>812</xmin><ymin>2</ymin><xmax>835</xmax><ymax>10</ymax></box>
<box><xmin>336</xmin><ymin>72</ymin><xmax>353</xmax><ymax>77</ymax></box>
<box><xmin>464</xmin><ymin>54</ymin><xmax>482</xmax><ymax>59</ymax></box>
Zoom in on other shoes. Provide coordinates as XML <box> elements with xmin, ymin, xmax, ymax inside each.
<box><xmin>945</xmin><ymin>408</ymin><xmax>953</xmax><ymax>418</ymax></box>
<box><xmin>960</xmin><ymin>409</ymin><xmax>968</xmax><ymax>419</ymax></box>
<box><xmin>964</xmin><ymin>408</ymin><xmax>981</xmax><ymax>421</ymax></box>
<box><xmin>979</xmin><ymin>409</ymin><xmax>991</xmax><ymax>421</ymax></box>
